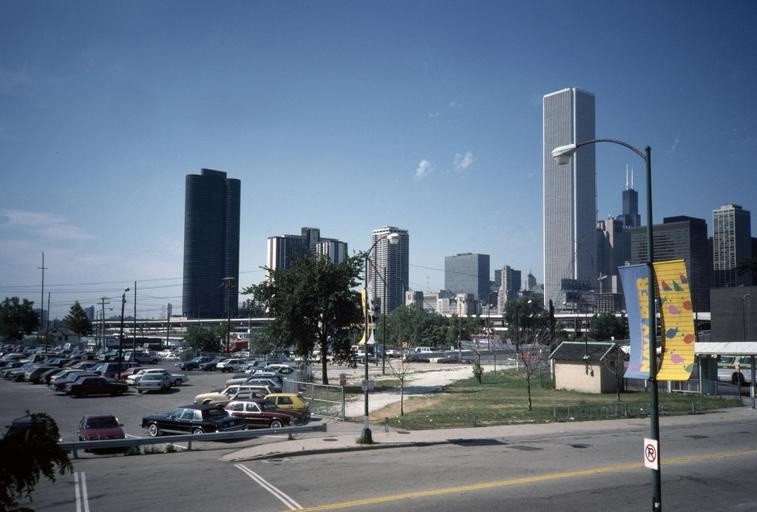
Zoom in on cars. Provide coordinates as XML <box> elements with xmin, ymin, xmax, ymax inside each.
<box><xmin>77</xmin><ymin>414</ymin><xmax>126</xmax><ymax>453</ymax></box>
<box><xmin>216</xmin><ymin>359</ymin><xmax>255</xmax><ymax>373</ymax></box>
<box><xmin>194</xmin><ymin>365</ymin><xmax>311</xmax><ymax>413</ymax></box>
<box><xmin>0</xmin><ymin>339</ymin><xmax>189</xmax><ymax>399</ymax></box>
<box><xmin>312</xmin><ymin>342</ymin><xmax>477</xmax><ymax>363</ymax></box>
<box><xmin>4</xmin><ymin>415</ymin><xmax>63</xmax><ymax>443</ymax></box>
<box><xmin>198</xmin><ymin>358</ymin><xmax>226</xmax><ymax>371</ymax></box>
<box><xmin>223</xmin><ymin>399</ymin><xmax>310</xmax><ymax>433</ymax></box>
<box><xmin>174</xmin><ymin>356</ymin><xmax>214</xmax><ymax>371</ymax></box>
<box><xmin>139</xmin><ymin>406</ymin><xmax>249</xmax><ymax>443</ymax></box>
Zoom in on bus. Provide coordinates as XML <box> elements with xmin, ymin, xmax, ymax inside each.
<box><xmin>716</xmin><ymin>356</ymin><xmax>757</xmax><ymax>384</ymax></box>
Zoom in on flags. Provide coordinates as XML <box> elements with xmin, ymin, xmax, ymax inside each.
<box><xmin>355</xmin><ymin>287</ymin><xmax>368</xmax><ymax>346</ymax></box>
<box><xmin>616</xmin><ymin>263</ymin><xmax>654</xmax><ymax>380</ymax></box>
<box><xmin>365</xmin><ymin>286</ymin><xmax>375</xmax><ymax>344</ymax></box>
<box><xmin>651</xmin><ymin>260</ymin><xmax>695</xmax><ymax>381</ymax></box>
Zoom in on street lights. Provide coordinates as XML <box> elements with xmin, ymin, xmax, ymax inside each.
<box><xmin>551</xmin><ymin>138</ymin><xmax>664</xmax><ymax>512</ymax></box>
<box><xmin>358</xmin><ymin>232</ymin><xmax>401</xmax><ymax>444</ymax></box>
<box><xmin>222</xmin><ymin>276</ymin><xmax>236</xmax><ymax>356</ymax></box>
<box><xmin>487</xmin><ymin>289</ymin><xmax>497</xmax><ymax>350</ymax></box>
<box><xmin>742</xmin><ymin>293</ymin><xmax>752</xmax><ymax>340</ymax></box>
<box><xmin>117</xmin><ymin>288</ymin><xmax>130</xmax><ymax>379</ymax></box>
